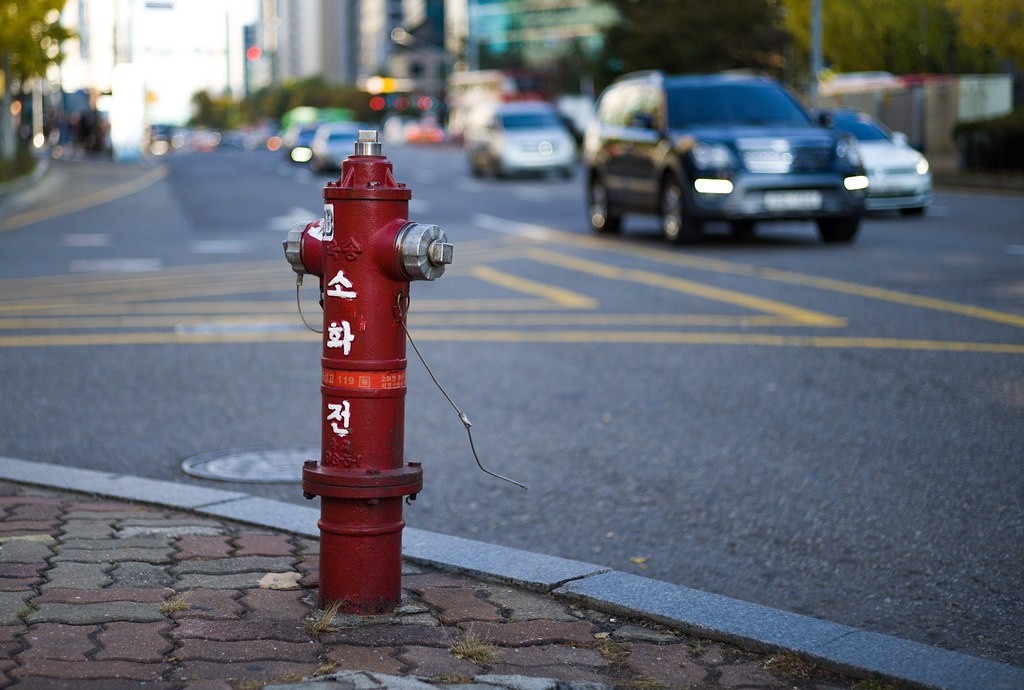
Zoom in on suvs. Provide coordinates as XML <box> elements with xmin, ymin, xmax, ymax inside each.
<box><xmin>584</xmin><ymin>68</ymin><xmax>868</xmax><ymax>244</ymax></box>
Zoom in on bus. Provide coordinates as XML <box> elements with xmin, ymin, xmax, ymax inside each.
<box><xmin>450</xmin><ymin>68</ymin><xmax>549</xmax><ymax>144</ymax></box>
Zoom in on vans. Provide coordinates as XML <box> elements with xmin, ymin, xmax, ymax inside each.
<box><xmin>463</xmin><ymin>103</ymin><xmax>578</xmax><ymax>182</ymax></box>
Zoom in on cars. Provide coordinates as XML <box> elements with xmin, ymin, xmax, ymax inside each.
<box><xmin>308</xmin><ymin>124</ymin><xmax>364</xmax><ymax>174</ymax></box>
<box><xmin>813</xmin><ymin>110</ymin><xmax>932</xmax><ymax>219</ymax></box>
<box><xmin>282</xmin><ymin>124</ymin><xmax>320</xmax><ymax>157</ymax></box>
<box><xmin>383</xmin><ymin>116</ymin><xmax>441</xmax><ymax>143</ymax></box>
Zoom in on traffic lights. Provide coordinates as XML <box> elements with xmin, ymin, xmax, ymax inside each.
<box><xmin>369</xmin><ymin>96</ymin><xmax>434</xmax><ymax>111</ymax></box>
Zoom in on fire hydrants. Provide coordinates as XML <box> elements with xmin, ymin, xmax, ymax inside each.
<box><xmin>280</xmin><ymin>127</ymin><xmax>453</xmax><ymax>615</ymax></box>
